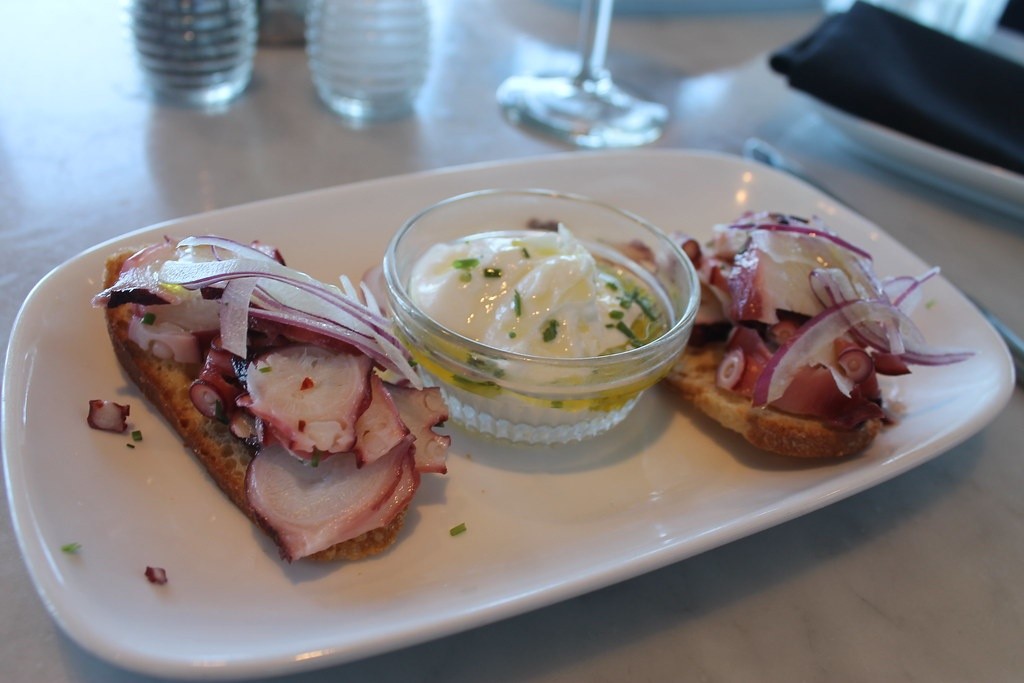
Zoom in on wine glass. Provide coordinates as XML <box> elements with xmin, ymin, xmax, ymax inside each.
<box><xmin>495</xmin><ymin>0</ymin><xmax>670</xmax><ymax>147</ymax></box>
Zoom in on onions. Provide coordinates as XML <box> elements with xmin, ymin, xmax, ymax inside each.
<box><xmin>632</xmin><ymin>211</ymin><xmax>976</xmax><ymax>412</ymax></box>
<box><xmin>156</xmin><ymin>233</ymin><xmax>421</xmax><ymax>386</ymax></box>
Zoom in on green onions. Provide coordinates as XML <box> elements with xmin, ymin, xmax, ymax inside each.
<box><xmin>449</xmin><ymin>245</ymin><xmax>664</xmax><ymax>411</ymax></box>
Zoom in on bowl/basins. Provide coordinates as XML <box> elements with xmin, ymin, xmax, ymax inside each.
<box><xmin>380</xmin><ymin>184</ymin><xmax>701</xmax><ymax>445</ymax></box>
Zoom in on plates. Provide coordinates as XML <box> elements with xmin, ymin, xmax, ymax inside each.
<box><xmin>0</xmin><ymin>148</ymin><xmax>1015</xmax><ymax>678</ymax></box>
<box><xmin>789</xmin><ymin>90</ymin><xmax>1024</xmax><ymax>218</ymax></box>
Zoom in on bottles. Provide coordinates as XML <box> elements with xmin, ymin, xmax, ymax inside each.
<box><xmin>306</xmin><ymin>0</ymin><xmax>438</xmax><ymax>125</ymax></box>
<box><xmin>130</xmin><ymin>1</ymin><xmax>259</xmax><ymax>111</ymax></box>
